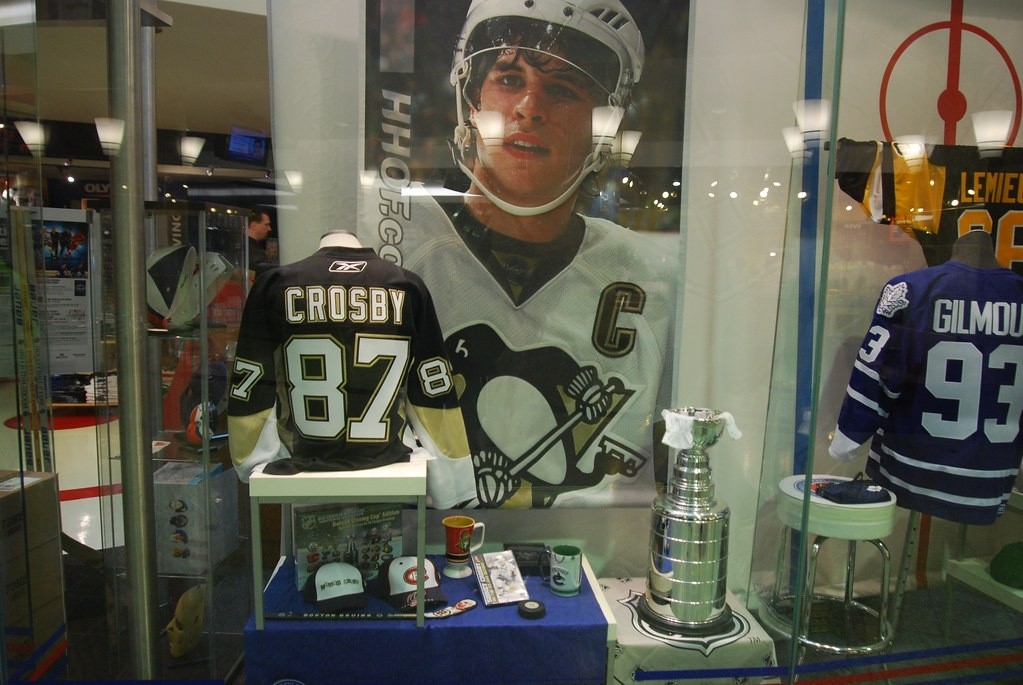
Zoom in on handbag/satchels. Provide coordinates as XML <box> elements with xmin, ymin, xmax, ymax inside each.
<box><xmin>815</xmin><ymin>472</ymin><xmax>892</xmax><ymax>504</ymax></box>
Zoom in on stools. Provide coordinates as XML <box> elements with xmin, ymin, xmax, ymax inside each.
<box><xmin>752</xmin><ymin>473</ymin><xmax>897</xmax><ymax>659</ymax></box>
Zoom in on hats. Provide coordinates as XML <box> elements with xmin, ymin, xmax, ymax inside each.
<box><xmin>378</xmin><ymin>555</ymin><xmax>448</xmax><ymax>614</ymax></box>
<box><xmin>301</xmin><ymin>562</ymin><xmax>368</xmax><ymax>613</ymax></box>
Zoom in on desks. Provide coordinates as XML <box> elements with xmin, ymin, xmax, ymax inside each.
<box><xmin>248</xmin><ymin>455</ymin><xmax>429</xmax><ymax>632</ymax></box>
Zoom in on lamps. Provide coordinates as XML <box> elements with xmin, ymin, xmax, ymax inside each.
<box><xmin>0</xmin><ymin>93</ymin><xmax>1023</xmax><ymax>205</ymax></box>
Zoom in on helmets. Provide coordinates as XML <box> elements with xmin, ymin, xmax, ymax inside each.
<box><xmin>145</xmin><ymin>245</ymin><xmax>196</xmax><ymax>329</ymax></box>
<box><xmin>450</xmin><ymin>0</ymin><xmax>645</xmax><ymax>119</ymax></box>
<box><xmin>188</xmin><ymin>251</ymin><xmax>235</xmax><ymax>322</ymax></box>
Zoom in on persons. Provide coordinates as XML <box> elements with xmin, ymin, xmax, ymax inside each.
<box><xmin>828</xmin><ymin>232</ymin><xmax>1023</xmax><ymax>527</ymax></box>
<box><xmin>358</xmin><ymin>0</ymin><xmax>677</xmax><ymax>510</ymax></box>
<box><xmin>33</xmin><ymin>225</ymin><xmax>86</xmax><ymax>278</ymax></box>
<box><xmin>235</xmin><ymin>209</ymin><xmax>271</xmax><ymax>279</ymax></box>
<box><xmin>227</xmin><ymin>230</ymin><xmax>477</xmax><ymax>511</ymax></box>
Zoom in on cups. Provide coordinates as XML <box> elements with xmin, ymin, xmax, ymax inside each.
<box><xmin>439</xmin><ymin>516</ymin><xmax>485</xmax><ymax>579</ymax></box>
<box><xmin>538</xmin><ymin>544</ymin><xmax>582</xmax><ymax>597</ymax></box>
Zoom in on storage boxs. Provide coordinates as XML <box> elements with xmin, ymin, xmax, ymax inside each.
<box><xmin>1</xmin><ymin>469</ymin><xmax>69</xmax><ymax>684</ymax></box>
<box><xmin>151</xmin><ymin>463</ymin><xmax>242</xmax><ymax>576</ymax></box>
